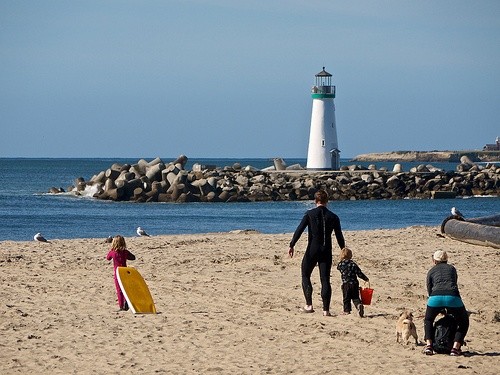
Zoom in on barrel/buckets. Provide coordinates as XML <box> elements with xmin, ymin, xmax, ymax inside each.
<box><xmin>359</xmin><ymin>281</ymin><xmax>374</xmax><ymax>305</ymax></box>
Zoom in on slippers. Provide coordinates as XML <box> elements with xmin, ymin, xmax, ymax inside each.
<box><xmin>300</xmin><ymin>307</ymin><xmax>313</xmax><ymax>313</ymax></box>
<box><xmin>323</xmin><ymin>313</ymin><xmax>331</xmax><ymax>316</ymax></box>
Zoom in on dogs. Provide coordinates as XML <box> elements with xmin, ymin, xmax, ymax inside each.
<box><xmin>395</xmin><ymin>310</ymin><xmax>419</xmax><ymax>347</ymax></box>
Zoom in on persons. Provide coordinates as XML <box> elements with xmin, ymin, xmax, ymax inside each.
<box><xmin>107</xmin><ymin>235</ymin><xmax>135</xmax><ymax>311</ymax></box>
<box><xmin>337</xmin><ymin>248</ymin><xmax>369</xmax><ymax>316</ymax></box>
<box><xmin>432</xmin><ymin>308</ymin><xmax>466</xmax><ymax>355</ymax></box>
<box><xmin>422</xmin><ymin>250</ymin><xmax>469</xmax><ymax>356</ymax></box>
<box><xmin>289</xmin><ymin>190</ymin><xmax>345</xmax><ymax>316</ymax></box>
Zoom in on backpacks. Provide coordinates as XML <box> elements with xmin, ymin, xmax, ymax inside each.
<box><xmin>432</xmin><ymin>315</ymin><xmax>458</xmax><ymax>354</ymax></box>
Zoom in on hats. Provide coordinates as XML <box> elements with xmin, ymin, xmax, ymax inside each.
<box><xmin>433</xmin><ymin>250</ymin><xmax>448</xmax><ymax>261</ymax></box>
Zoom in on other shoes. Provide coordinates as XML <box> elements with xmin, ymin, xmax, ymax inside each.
<box><xmin>342</xmin><ymin>311</ymin><xmax>351</xmax><ymax>315</ymax></box>
<box><xmin>425</xmin><ymin>345</ymin><xmax>433</xmax><ymax>355</ymax></box>
<box><xmin>358</xmin><ymin>304</ymin><xmax>364</xmax><ymax>317</ymax></box>
<box><xmin>450</xmin><ymin>348</ymin><xmax>462</xmax><ymax>355</ymax></box>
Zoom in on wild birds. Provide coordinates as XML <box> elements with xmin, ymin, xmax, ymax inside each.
<box><xmin>449</xmin><ymin>206</ymin><xmax>465</xmax><ymax>219</ymax></box>
<box><xmin>136</xmin><ymin>225</ymin><xmax>150</xmax><ymax>237</ymax></box>
<box><xmin>34</xmin><ymin>233</ymin><xmax>52</xmax><ymax>244</ymax></box>
